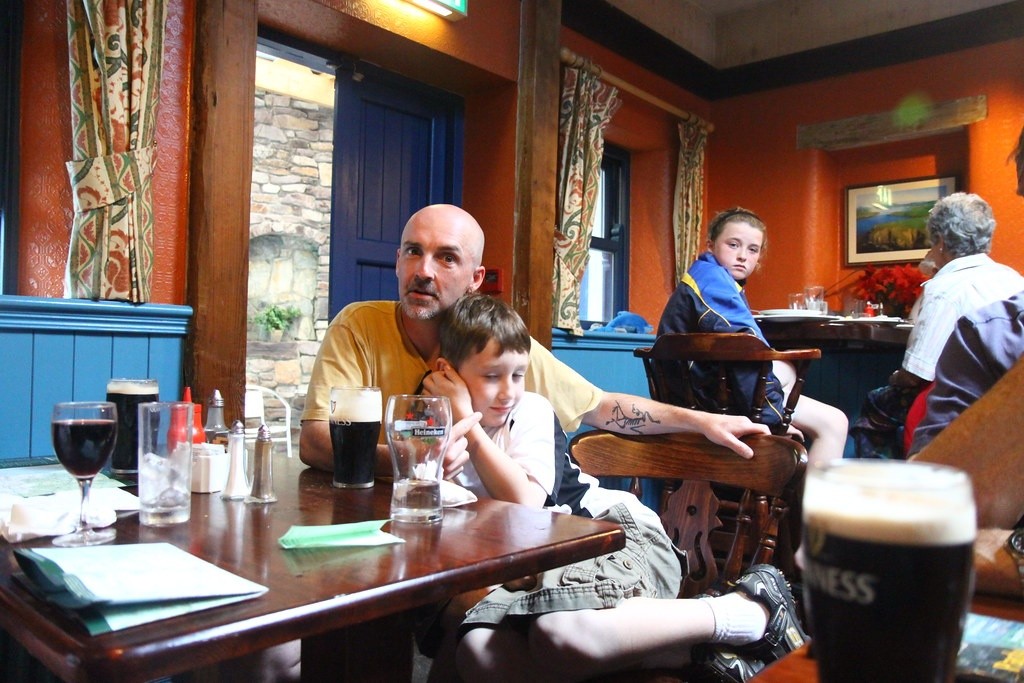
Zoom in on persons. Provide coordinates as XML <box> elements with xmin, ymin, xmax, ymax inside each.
<box><xmin>408</xmin><ymin>302</ymin><xmax>810</xmax><ymax>683</ymax></box>
<box><xmin>850</xmin><ymin>128</ymin><xmax>1024</xmax><ymax>601</ymax></box>
<box><xmin>654</xmin><ymin>208</ymin><xmax>848</xmax><ymax>513</ymax></box>
<box><xmin>300</xmin><ymin>203</ymin><xmax>772</xmax><ymax>683</ymax></box>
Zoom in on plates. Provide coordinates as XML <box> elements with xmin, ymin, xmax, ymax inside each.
<box><xmin>759</xmin><ymin>308</ymin><xmax>839</xmax><ymax>322</ymax></box>
<box><xmin>829</xmin><ymin>319</ymin><xmax>904</xmax><ymax>323</ymax></box>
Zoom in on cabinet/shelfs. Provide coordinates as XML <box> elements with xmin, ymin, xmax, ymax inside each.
<box><xmin>553</xmin><ymin>327</ymin><xmax>661</xmax><ymax>513</ymax></box>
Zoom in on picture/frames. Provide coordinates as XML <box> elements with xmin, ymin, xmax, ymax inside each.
<box><xmin>845</xmin><ymin>171</ymin><xmax>959</xmax><ymax>266</ymax></box>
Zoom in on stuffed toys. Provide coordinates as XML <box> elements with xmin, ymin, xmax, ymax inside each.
<box><xmin>589</xmin><ymin>308</ymin><xmax>651</xmax><ymax>333</ymax></box>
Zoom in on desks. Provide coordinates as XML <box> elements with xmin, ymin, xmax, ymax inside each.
<box><xmin>754</xmin><ymin>316</ymin><xmax>911</xmax><ymax>347</ymax></box>
<box><xmin>0</xmin><ymin>480</ymin><xmax>627</xmax><ymax>683</ymax></box>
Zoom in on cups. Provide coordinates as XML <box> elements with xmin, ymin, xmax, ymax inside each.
<box><xmin>788</xmin><ymin>293</ymin><xmax>806</xmax><ymax>310</ymax></box>
<box><xmin>136</xmin><ymin>401</ymin><xmax>194</xmax><ymax>526</ymax></box>
<box><xmin>329</xmin><ymin>385</ymin><xmax>383</xmax><ymax>489</ymax></box>
<box><xmin>803</xmin><ymin>286</ymin><xmax>824</xmax><ymax>311</ymax></box>
<box><xmin>853</xmin><ymin>300</ymin><xmax>866</xmax><ymax>319</ymax></box>
<box><xmin>384</xmin><ymin>393</ymin><xmax>453</xmax><ymax>524</ymax></box>
<box><xmin>799</xmin><ymin>456</ymin><xmax>978</xmax><ymax>683</ymax></box>
<box><xmin>105</xmin><ymin>378</ymin><xmax>160</xmax><ymax>475</ymax></box>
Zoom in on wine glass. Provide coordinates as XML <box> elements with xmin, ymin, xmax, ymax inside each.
<box><xmin>51</xmin><ymin>402</ymin><xmax>119</xmax><ymax>548</ymax></box>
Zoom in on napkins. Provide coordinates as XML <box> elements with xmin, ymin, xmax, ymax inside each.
<box><xmin>396</xmin><ymin>477</ymin><xmax>477</xmax><ymax>508</ymax></box>
<box><xmin>0</xmin><ymin>489</ymin><xmax>120</xmax><ymax>543</ymax></box>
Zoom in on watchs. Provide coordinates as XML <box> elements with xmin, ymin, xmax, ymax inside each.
<box><xmin>1002</xmin><ymin>528</ymin><xmax>1024</xmax><ymax>591</ymax></box>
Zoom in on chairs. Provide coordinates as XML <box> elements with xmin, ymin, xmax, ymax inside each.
<box><xmin>565</xmin><ymin>331</ymin><xmax>1024</xmax><ymax>683</ymax></box>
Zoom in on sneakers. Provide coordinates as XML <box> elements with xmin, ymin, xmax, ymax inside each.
<box><xmin>687</xmin><ymin>587</ymin><xmax>767</xmax><ymax>683</ymax></box>
<box><xmin>726</xmin><ymin>563</ymin><xmax>811</xmax><ymax>665</ymax></box>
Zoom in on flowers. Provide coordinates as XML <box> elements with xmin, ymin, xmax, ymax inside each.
<box><xmin>859</xmin><ymin>263</ymin><xmax>930</xmax><ymax>317</ymax></box>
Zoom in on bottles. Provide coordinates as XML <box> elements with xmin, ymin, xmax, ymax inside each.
<box><xmin>250</xmin><ymin>424</ymin><xmax>280</xmax><ymax>503</ymax></box>
<box><xmin>203</xmin><ymin>390</ymin><xmax>229</xmax><ymax>454</ymax></box>
<box><xmin>168</xmin><ymin>386</ymin><xmax>205</xmax><ymax>455</ymax></box>
<box><xmin>219</xmin><ymin>420</ymin><xmax>250</xmax><ymax>501</ymax></box>
<box><xmin>865</xmin><ymin>301</ymin><xmax>875</xmax><ymax>318</ymax></box>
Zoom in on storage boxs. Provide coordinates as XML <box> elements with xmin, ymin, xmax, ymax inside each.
<box><xmin>0</xmin><ymin>294</ymin><xmax>195</xmax><ymax>466</ymax></box>
